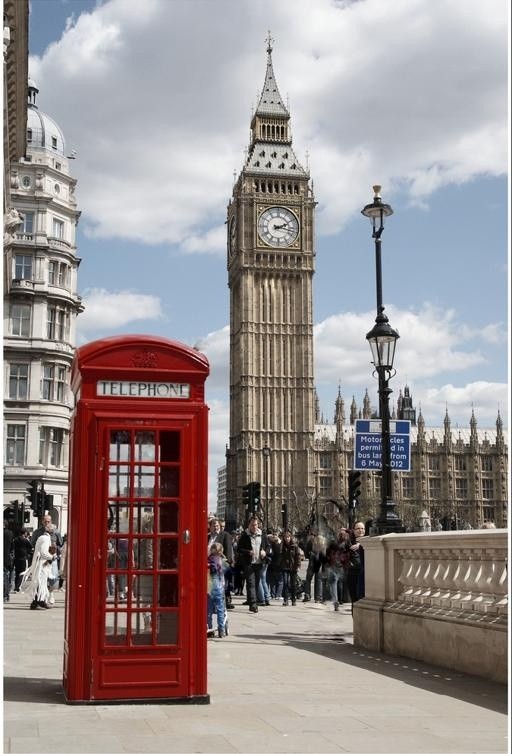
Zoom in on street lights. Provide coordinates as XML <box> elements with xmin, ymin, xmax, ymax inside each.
<box><xmin>262</xmin><ymin>441</ymin><xmax>271</xmax><ymax>529</ymax></box>
<box><xmin>361</xmin><ymin>185</ymin><xmax>406</xmax><ymax>534</ymax></box>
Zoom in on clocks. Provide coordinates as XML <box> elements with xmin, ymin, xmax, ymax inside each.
<box><xmin>257</xmin><ymin>205</ymin><xmax>300</xmax><ymax>248</ymax></box>
<box><xmin>229</xmin><ymin>215</ymin><xmax>237</xmax><ymax>258</ymax></box>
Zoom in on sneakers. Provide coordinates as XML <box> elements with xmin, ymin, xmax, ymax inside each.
<box><xmin>226</xmin><ymin>604</ymin><xmax>235</xmax><ymax>609</ymax></box>
<box><xmin>242</xmin><ymin>594</ymin><xmax>340</xmax><ymax>614</ymax></box>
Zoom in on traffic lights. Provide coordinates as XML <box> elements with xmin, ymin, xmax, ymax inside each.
<box><xmin>243</xmin><ymin>482</ymin><xmax>260</xmax><ymax>513</ymax></box>
<box><xmin>349</xmin><ymin>470</ymin><xmax>361</xmax><ymax>508</ymax></box>
<box><xmin>26</xmin><ymin>480</ymin><xmax>44</xmax><ymax>517</ymax></box>
<box><xmin>11</xmin><ymin>499</ymin><xmax>24</xmax><ymax>529</ymax></box>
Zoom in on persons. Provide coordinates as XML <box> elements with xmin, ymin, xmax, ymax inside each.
<box><xmin>107</xmin><ymin>516</ymin><xmax>153</xmax><ymax>630</ymax></box>
<box><xmin>209</xmin><ymin>514</ymin><xmax>305</xmax><ymax>639</ymax></box>
<box><xmin>481</xmin><ymin>522</ymin><xmax>496</xmax><ymax>529</ymax></box>
<box><xmin>3</xmin><ymin>514</ymin><xmax>68</xmax><ymax>610</ymax></box>
<box><xmin>299</xmin><ymin>521</ymin><xmax>364</xmax><ymax>619</ymax></box>
